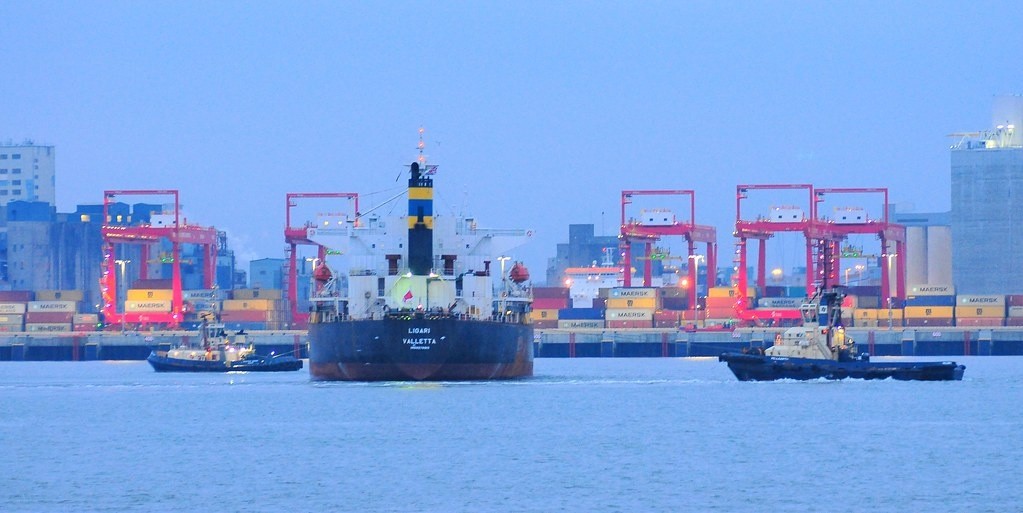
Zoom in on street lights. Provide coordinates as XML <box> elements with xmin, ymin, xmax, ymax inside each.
<box><xmin>689</xmin><ymin>255</ymin><xmax>705</xmax><ymax>330</ymax></box>
<box><xmin>114</xmin><ymin>259</ymin><xmax>131</xmax><ymax>335</ymax></box>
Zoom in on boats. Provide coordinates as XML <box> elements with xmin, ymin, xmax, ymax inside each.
<box><xmin>719</xmin><ymin>280</ymin><xmax>967</xmax><ymax>382</ymax></box>
<box><xmin>510</xmin><ymin>263</ymin><xmax>529</xmax><ymax>283</ymax></box>
<box><xmin>145</xmin><ymin>311</ymin><xmax>303</xmax><ymax>372</ymax></box>
<box><xmin>301</xmin><ymin>124</ymin><xmax>536</xmax><ymax>386</ymax></box>
<box><xmin>313</xmin><ymin>262</ymin><xmax>332</xmax><ymax>281</ymax></box>
